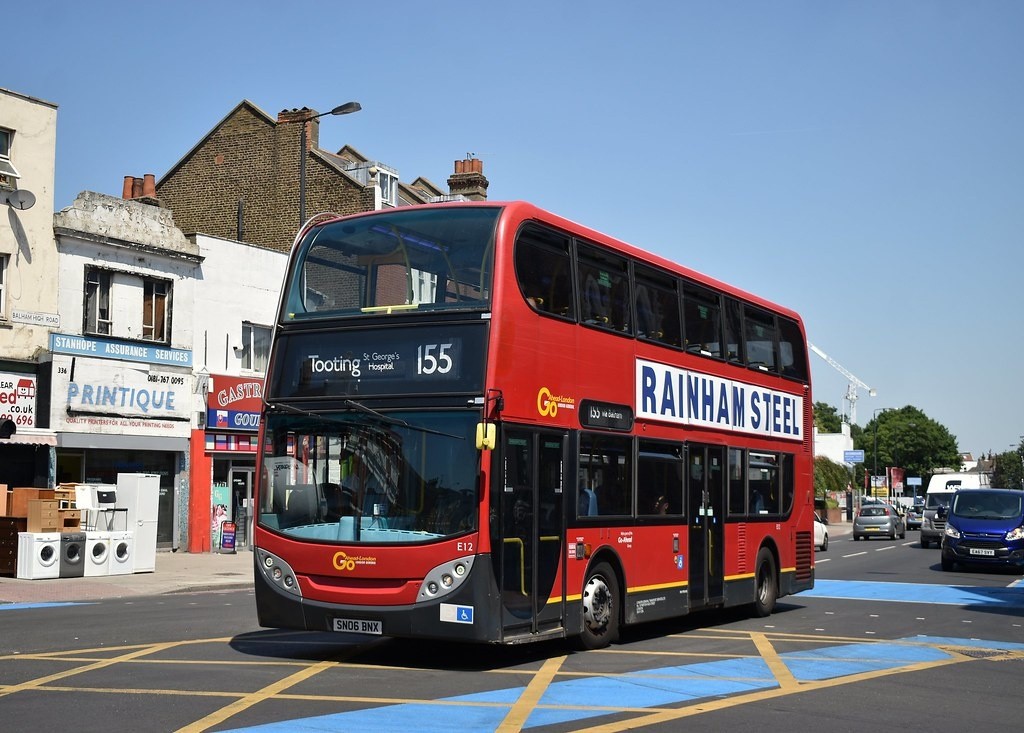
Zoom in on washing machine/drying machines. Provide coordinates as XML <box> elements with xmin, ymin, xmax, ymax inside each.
<box><xmin>16</xmin><ymin>531</ymin><xmax>135</xmax><ymax>580</ymax></box>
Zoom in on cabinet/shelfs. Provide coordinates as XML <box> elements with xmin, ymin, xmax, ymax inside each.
<box><xmin>12</xmin><ymin>486</ymin><xmax>82</xmax><ymax>533</ymax></box>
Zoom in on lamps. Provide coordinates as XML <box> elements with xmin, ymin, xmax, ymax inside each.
<box><xmin>204</xmin><ymin>378</ymin><xmax>214</xmax><ymax>393</ymax></box>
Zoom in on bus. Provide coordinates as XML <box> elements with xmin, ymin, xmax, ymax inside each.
<box><xmin>252</xmin><ymin>203</ymin><xmax>819</xmax><ymax>649</ymax></box>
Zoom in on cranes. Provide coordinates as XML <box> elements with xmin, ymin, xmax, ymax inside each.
<box><xmin>807</xmin><ymin>339</ymin><xmax>878</xmax><ymax>424</ymax></box>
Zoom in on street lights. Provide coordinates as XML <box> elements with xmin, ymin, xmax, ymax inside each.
<box><xmin>299</xmin><ymin>102</ymin><xmax>361</xmax><ymax>226</ymax></box>
<box><xmin>894</xmin><ymin>423</ymin><xmax>917</xmax><ymax>508</ymax></box>
<box><xmin>874</xmin><ymin>408</ymin><xmax>901</xmax><ymax>501</ymax></box>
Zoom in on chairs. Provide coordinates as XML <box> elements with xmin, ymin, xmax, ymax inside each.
<box><xmin>275</xmin><ymin>485</ymin><xmax>322</xmax><ymax>529</ymax></box>
<box><xmin>572</xmin><ymin>485</ymin><xmax>785</xmax><ymax>516</ymax></box>
<box><xmin>529</xmin><ymin>296</ymin><xmax>775</xmax><ymax>371</ymax></box>
<box><xmin>95</xmin><ymin>490</ymin><xmax>128</xmax><ymax>530</ymax></box>
<box><xmin>865</xmin><ymin>510</ymin><xmax>872</xmax><ymax>515</ymax></box>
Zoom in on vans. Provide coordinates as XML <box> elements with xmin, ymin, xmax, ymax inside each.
<box><xmin>937</xmin><ymin>489</ymin><xmax>1024</xmax><ymax>574</ymax></box>
<box><xmin>918</xmin><ymin>473</ymin><xmax>992</xmax><ymax>549</ymax></box>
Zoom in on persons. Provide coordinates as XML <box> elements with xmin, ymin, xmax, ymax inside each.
<box><xmin>213</xmin><ymin>503</ymin><xmax>228</xmax><ymax>532</ymax></box>
<box><xmin>645</xmin><ymin>493</ymin><xmax>669</xmax><ymax>516</ymax></box>
<box><xmin>1001</xmin><ymin>495</ymin><xmax>1021</xmax><ymax>517</ymax></box>
<box><xmin>334</xmin><ymin>441</ymin><xmax>390</xmax><ymax>515</ymax></box>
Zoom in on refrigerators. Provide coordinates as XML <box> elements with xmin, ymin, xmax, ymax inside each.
<box><xmin>113</xmin><ymin>473</ymin><xmax>161</xmax><ymax>573</ymax></box>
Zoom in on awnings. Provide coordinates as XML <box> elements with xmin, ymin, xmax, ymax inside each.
<box><xmin>0</xmin><ymin>426</ymin><xmax>58</xmax><ymax>447</ymax></box>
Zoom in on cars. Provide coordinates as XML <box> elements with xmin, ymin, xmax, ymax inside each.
<box><xmin>906</xmin><ymin>505</ymin><xmax>925</xmax><ymax>530</ymax></box>
<box><xmin>853</xmin><ymin>504</ymin><xmax>906</xmax><ymax>540</ymax></box>
<box><xmin>814</xmin><ymin>511</ymin><xmax>829</xmax><ymax>551</ymax></box>
